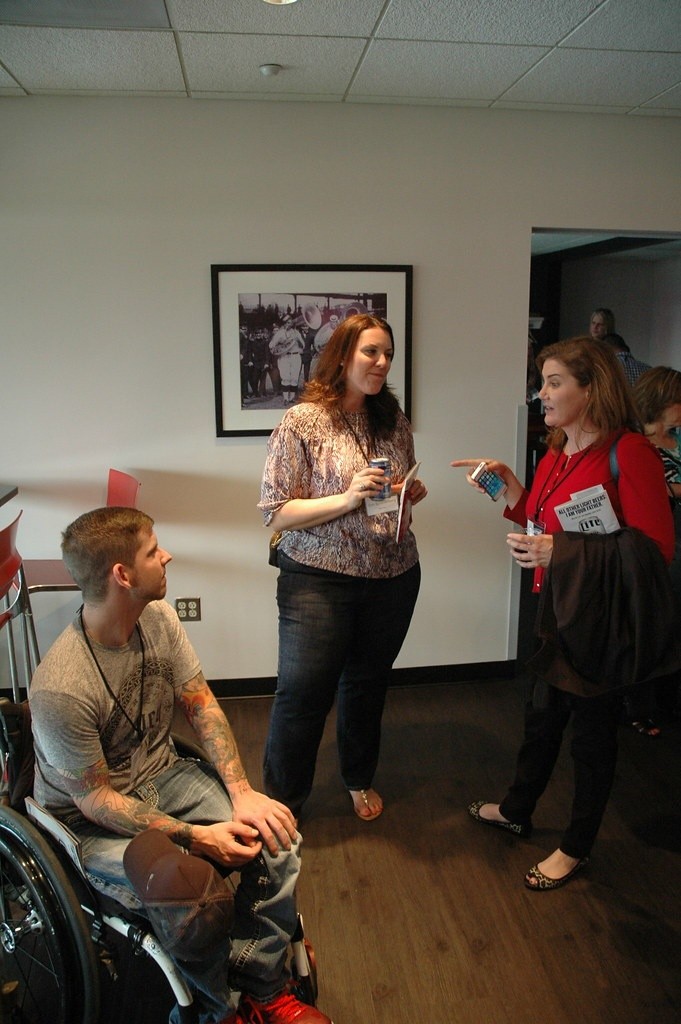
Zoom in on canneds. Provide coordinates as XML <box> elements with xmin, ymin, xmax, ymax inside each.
<box><xmin>368</xmin><ymin>457</ymin><xmax>391</xmax><ymax>500</ymax></box>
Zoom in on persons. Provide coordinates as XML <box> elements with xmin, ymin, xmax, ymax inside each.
<box><xmin>630</xmin><ymin>364</ymin><xmax>681</xmax><ymax>736</ymax></box>
<box><xmin>31</xmin><ymin>506</ymin><xmax>302</xmax><ymax>1024</ymax></box>
<box><xmin>238</xmin><ymin>303</ymin><xmax>383</xmax><ymax>407</ymax></box>
<box><xmin>585</xmin><ymin>307</ymin><xmax>616</xmax><ymax>341</ymax></box>
<box><xmin>448</xmin><ymin>338</ymin><xmax>674</xmax><ymax>893</ymax></box>
<box><xmin>604</xmin><ymin>332</ymin><xmax>653</xmax><ymax>390</ymax></box>
<box><xmin>258</xmin><ymin>317</ymin><xmax>428</xmax><ymax>822</ymax></box>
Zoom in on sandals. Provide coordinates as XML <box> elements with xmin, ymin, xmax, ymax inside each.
<box><xmin>348</xmin><ymin>786</ymin><xmax>384</xmax><ymax>821</ymax></box>
<box><xmin>626</xmin><ymin>713</ymin><xmax>663</xmax><ymax>740</ymax></box>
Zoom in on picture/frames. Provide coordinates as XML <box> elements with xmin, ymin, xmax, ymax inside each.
<box><xmin>210</xmin><ymin>264</ymin><xmax>413</xmax><ymax>438</ymax></box>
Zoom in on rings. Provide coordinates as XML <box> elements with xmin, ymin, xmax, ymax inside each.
<box><xmin>360</xmin><ymin>482</ymin><xmax>365</xmax><ymax>489</ymax></box>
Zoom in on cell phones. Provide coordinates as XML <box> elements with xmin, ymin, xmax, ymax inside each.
<box><xmin>471</xmin><ymin>462</ymin><xmax>508</xmax><ymax>501</ymax></box>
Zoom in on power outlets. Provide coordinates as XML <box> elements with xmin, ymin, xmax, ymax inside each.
<box><xmin>176</xmin><ymin>597</ymin><xmax>201</xmax><ymax>622</ymax></box>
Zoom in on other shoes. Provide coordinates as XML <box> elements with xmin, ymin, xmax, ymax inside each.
<box><xmin>525</xmin><ymin>845</ymin><xmax>590</xmax><ymax>891</ymax></box>
<box><xmin>467</xmin><ymin>797</ymin><xmax>535</xmax><ymax>837</ymax></box>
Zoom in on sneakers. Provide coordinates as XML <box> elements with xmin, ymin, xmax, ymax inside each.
<box><xmin>217</xmin><ymin>987</ymin><xmax>334</xmax><ymax>1024</ymax></box>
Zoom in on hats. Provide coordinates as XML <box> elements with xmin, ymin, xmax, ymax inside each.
<box><xmin>124</xmin><ymin>830</ymin><xmax>237</xmax><ymax>961</ymax></box>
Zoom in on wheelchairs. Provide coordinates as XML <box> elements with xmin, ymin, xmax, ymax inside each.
<box><xmin>0</xmin><ymin>695</ymin><xmax>320</xmax><ymax>1024</ymax></box>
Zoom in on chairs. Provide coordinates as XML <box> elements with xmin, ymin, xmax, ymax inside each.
<box><xmin>0</xmin><ymin>509</ymin><xmax>32</xmax><ymax>707</ymax></box>
<box><xmin>3</xmin><ymin>467</ymin><xmax>140</xmax><ymax>706</ymax></box>
